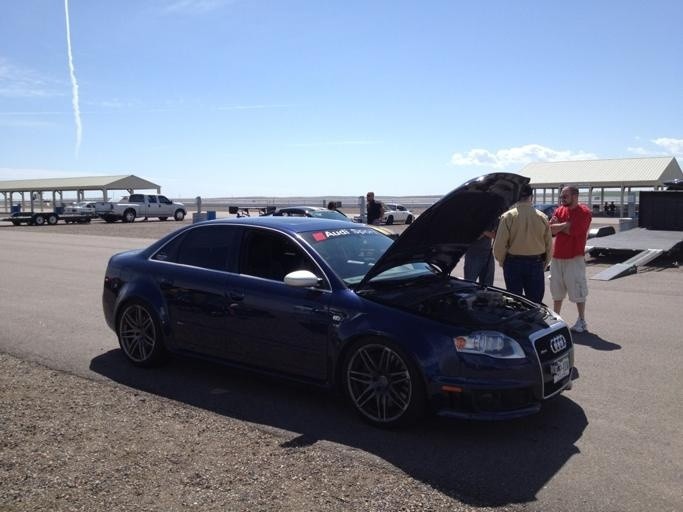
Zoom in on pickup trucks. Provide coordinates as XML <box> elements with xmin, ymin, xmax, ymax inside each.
<box><xmin>95</xmin><ymin>193</ymin><xmax>187</xmax><ymax>222</ymax></box>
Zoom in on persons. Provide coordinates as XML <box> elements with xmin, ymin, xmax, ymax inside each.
<box><xmin>608</xmin><ymin>202</ymin><xmax>616</xmax><ymax>217</ymax></box>
<box><xmin>244</xmin><ymin>208</ymin><xmax>251</xmax><ymax>216</ymax></box>
<box><xmin>236</xmin><ymin>213</ymin><xmax>246</xmax><ymax>217</ymax></box>
<box><xmin>548</xmin><ymin>187</ymin><xmax>592</xmax><ymax>333</ymax></box>
<box><xmin>327</xmin><ymin>201</ymin><xmax>347</xmax><ymax>217</ymax></box>
<box><xmin>603</xmin><ymin>201</ymin><xmax>610</xmax><ymax>215</ymax></box>
<box><xmin>464</xmin><ymin>217</ymin><xmax>498</xmax><ymax>287</ymax></box>
<box><xmin>493</xmin><ymin>183</ymin><xmax>553</xmax><ymax>304</ymax></box>
<box><xmin>366</xmin><ymin>192</ymin><xmax>384</xmax><ymax>226</ymax></box>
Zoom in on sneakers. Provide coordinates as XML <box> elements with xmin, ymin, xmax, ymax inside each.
<box><xmin>570</xmin><ymin>320</ymin><xmax>588</xmax><ymax>332</ymax></box>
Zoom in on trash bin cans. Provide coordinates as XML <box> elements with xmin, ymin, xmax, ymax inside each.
<box><xmin>207</xmin><ymin>211</ymin><xmax>216</xmax><ymax>220</ymax></box>
<box><xmin>11</xmin><ymin>206</ymin><xmax>20</xmax><ymax>213</ymax></box>
<box><xmin>56</xmin><ymin>207</ymin><xmax>64</xmax><ymax>214</ymax></box>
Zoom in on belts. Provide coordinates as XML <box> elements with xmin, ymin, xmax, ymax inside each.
<box><xmin>507</xmin><ymin>253</ymin><xmax>545</xmax><ymax>260</ymax></box>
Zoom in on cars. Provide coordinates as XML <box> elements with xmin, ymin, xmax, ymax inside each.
<box><xmin>531</xmin><ymin>203</ymin><xmax>559</xmax><ymax>237</ymax></box>
<box><xmin>64</xmin><ymin>200</ymin><xmax>95</xmax><ymax>216</ymax></box>
<box><xmin>273</xmin><ymin>203</ymin><xmax>414</xmax><ymax>241</ymax></box>
<box><xmin>103</xmin><ymin>172</ymin><xmax>579</xmax><ymax>430</ymax></box>
